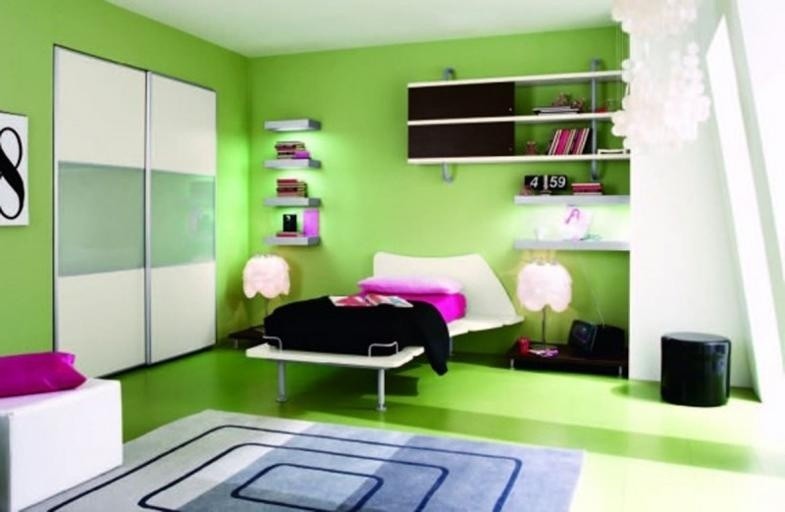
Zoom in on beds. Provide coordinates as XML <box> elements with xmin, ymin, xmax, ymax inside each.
<box><xmin>245</xmin><ymin>250</ymin><xmax>528</xmax><ymax>418</ymax></box>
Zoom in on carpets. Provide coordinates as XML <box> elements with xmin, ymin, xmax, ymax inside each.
<box><xmin>8</xmin><ymin>408</ymin><xmax>587</xmax><ymax>512</ymax></box>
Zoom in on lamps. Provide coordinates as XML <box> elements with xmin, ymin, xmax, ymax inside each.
<box><xmin>237</xmin><ymin>251</ymin><xmax>295</xmax><ymax>328</ymax></box>
<box><xmin>517</xmin><ymin>259</ymin><xmax>572</xmax><ymax>352</ymax></box>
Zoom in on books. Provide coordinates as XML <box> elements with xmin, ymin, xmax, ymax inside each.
<box><xmin>274</xmin><ymin>140</ymin><xmax>308</xmax><ymax>239</ymax></box>
<box><xmin>531</xmin><ymin>104</ymin><xmax>605</xmax><ymax>196</ymax></box>
<box><xmin>328</xmin><ymin>291</ymin><xmax>415</xmax><ymax>308</ymax></box>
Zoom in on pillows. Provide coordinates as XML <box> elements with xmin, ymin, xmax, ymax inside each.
<box><xmin>0</xmin><ymin>352</ymin><xmax>86</xmax><ymax>400</ymax></box>
<box><xmin>356</xmin><ymin>271</ymin><xmax>464</xmax><ymax>298</ymax></box>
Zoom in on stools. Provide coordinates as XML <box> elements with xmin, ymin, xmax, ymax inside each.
<box><xmin>659</xmin><ymin>326</ymin><xmax>732</xmax><ymax>407</ymax></box>
<box><xmin>0</xmin><ymin>376</ymin><xmax>126</xmax><ymax>512</ymax></box>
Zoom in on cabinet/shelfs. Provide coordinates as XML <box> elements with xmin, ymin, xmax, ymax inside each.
<box><xmin>401</xmin><ymin>67</ymin><xmax>630</xmax><ymax>166</ymax></box>
<box><xmin>262</xmin><ymin>116</ymin><xmax>324</xmax><ymax>248</ymax></box>
<box><xmin>228</xmin><ymin>324</ymin><xmax>268</xmax><ymax>350</ymax></box>
<box><xmin>507</xmin><ymin>338</ymin><xmax>626</xmax><ymax>379</ymax></box>
<box><xmin>512</xmin><ymin>185</ymin><xmax>632</xmax><ymax>254</ymax></box>
